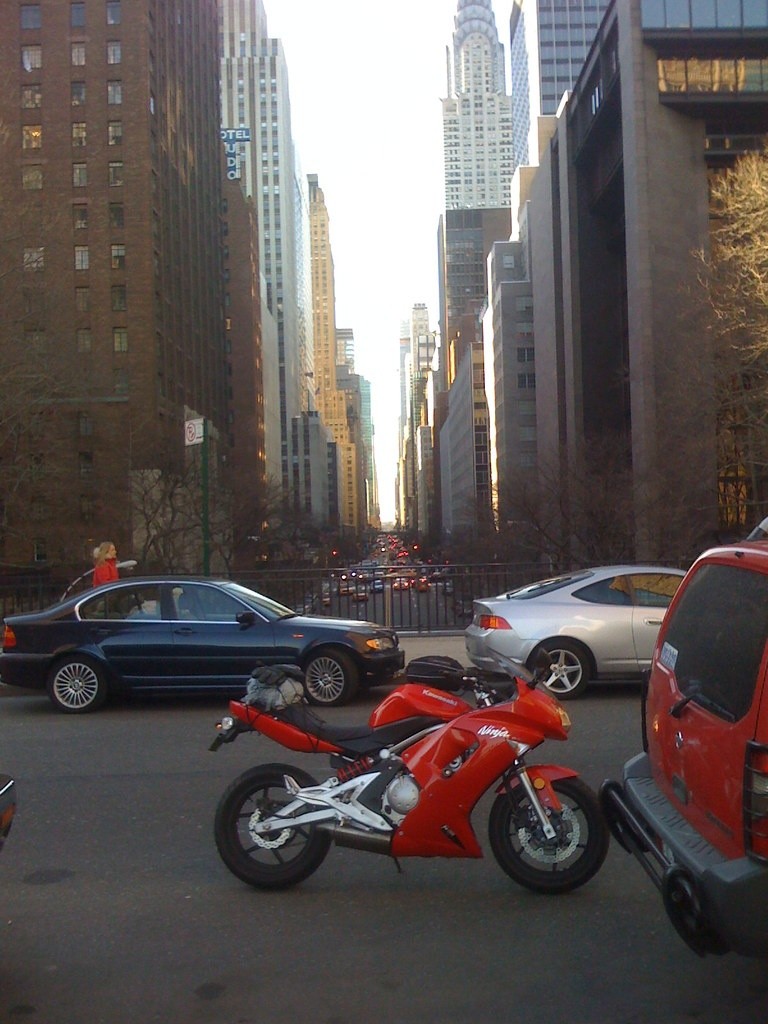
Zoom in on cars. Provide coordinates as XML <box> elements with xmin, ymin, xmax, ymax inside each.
<box><xmin>1</xmin><ymin>576</ymin><xmax>408</xmax><ymax>707</ymax></box>
<box><xmin>463</xmin><ymin>565</ymin><xmax>761</xmax><ymax>702</ymax></box>
<box><xmin>321</xmin><ymin>535</ymin><xmax>455</xmax><ymax>605</ymax></box>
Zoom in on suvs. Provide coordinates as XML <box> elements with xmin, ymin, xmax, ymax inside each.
<box><xmin>598</xmin><ymin>521</ymin><xmax>768</xmax><ymax>965</ymax></box>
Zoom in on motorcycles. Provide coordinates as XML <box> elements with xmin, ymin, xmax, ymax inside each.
<box><xmin>206</xmin><ymin>657</ymin><xmax>614</xmax><ymax>895</ymax></box>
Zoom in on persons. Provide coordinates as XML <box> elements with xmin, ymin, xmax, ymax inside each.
<box><xmin>93</xmin><ymin>542</ymin><xmax>118</xmax><ymax>588</ymax></box>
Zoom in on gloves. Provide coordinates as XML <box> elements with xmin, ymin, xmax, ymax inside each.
<box><xmin>252</xmin><ymin>664</ymin><xmax>304</xmax><ymax>685</ymax></box>
